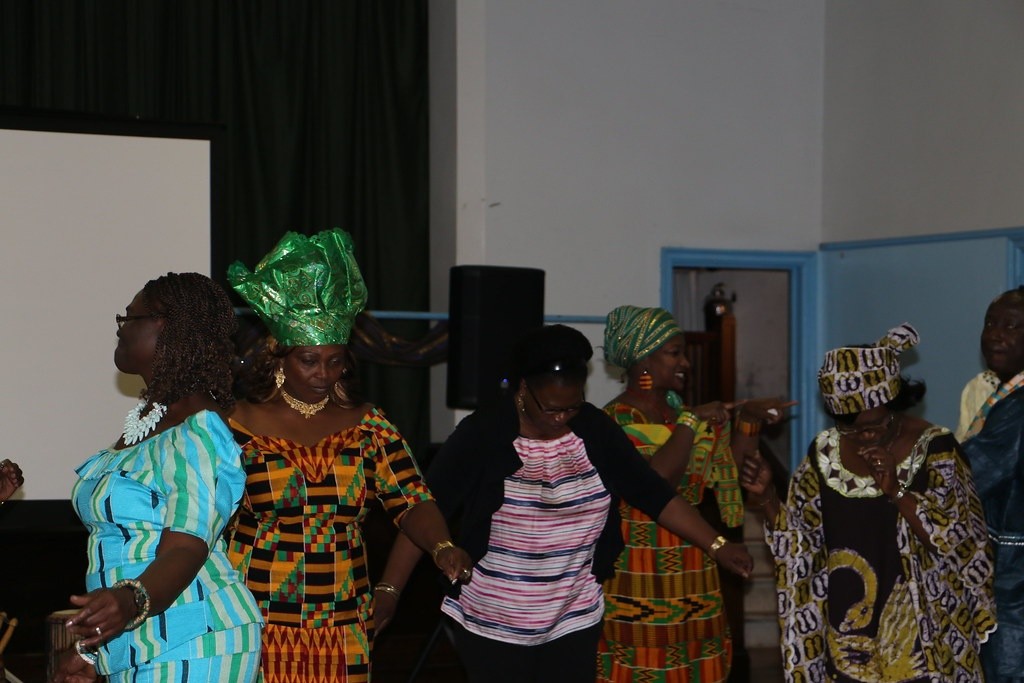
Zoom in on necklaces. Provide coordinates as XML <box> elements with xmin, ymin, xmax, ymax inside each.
<box><xmin>280</xmin><ymin>386</ymin><xmax>329</xmax><ymax>419</ymax></box>
<box><xmin>885</xmin><ymin>421</ymin><xmax>902</xmax><ymax>452</ymax></box>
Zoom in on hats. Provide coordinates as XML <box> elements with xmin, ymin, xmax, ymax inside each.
<box><xmin>822</xmin><ymin>321</ymin><xmax>922</xmax><ymax>415</ymax></box>
<box><xmin>603</xmin><ymin>306</ymin><xmax>684</xmax><ymax>369</ymax></box>
<box><xmin>226</xmin><ymin>226</ymin><xmax>368</xmax><ymax>346</ymax></box>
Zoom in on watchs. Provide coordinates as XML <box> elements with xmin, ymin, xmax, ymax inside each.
<box><xmin>124</xmin><ymin>584</ymin><xmax>145</xmax><ymax>616</ymax></box>
<box><xmin>892</xmin><ymin>483</ymin><xmax>908</xmax><ymax>500</ymax></box>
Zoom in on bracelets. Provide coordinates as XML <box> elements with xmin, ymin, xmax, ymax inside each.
<box><xmin>373</xmin><ymin>583</ymin><xmax>400</xmax><ymax>600</ymax></box>
<box><xmin>75</xmin><ymin>639</ymin><xmax>97</xmax><ymax>665</ymax></box>
<box><xmin>734</xmin><ymin>411</ymin><xmax>762</xmax><ymax>435</ymax></box>
<box><xmin>707</xmin><ymin>536</ymin><xmax>728</xmax><ymax>559</ymax></box>
<box><xmin>431</xmin><ymin>541</ymin><xmax>453</xmax><ymax>564</ymax></box>
<box><xmin>112</xmin><ymin>579</ymin><xmax>150</xmax><ymax>630</ymax></box>
<box><xmin>676</xmin><ymin>411</ymin><xmax>701</xmax><ymax>433</ymax></box>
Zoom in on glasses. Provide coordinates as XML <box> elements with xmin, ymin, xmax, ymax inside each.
<box><xmin>522</xmin><ymin>379</ymin><xmax>585</xmax><ymax>415</ymax></box>
<box><xmin>115</xmin><ymin>312</ymin><xmax>168</xmax><ymax>330</ymax></box>
<box><xmin>832</xmin><ymin>407</ymin><xmax>898</xmax><ymax>436</ymax></box>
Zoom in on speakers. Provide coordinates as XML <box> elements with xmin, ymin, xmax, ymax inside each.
<box><xmin>446</xmin><ymin>266</ymin><xmax>546</xmax><ymax>410</ymax></box>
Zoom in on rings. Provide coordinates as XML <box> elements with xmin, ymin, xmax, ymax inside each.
<box><xmin>877</xmin><ymin>459</ymin><xmax>880</xmax><ymax>465</ymax></box>
<box><xmin>95</xmin><ymin>627</ymin><xmax>101</xmax><ymax>634</ymax></box>
<box><xmin>462</xmin><ymin>568</ymin><xmax>470</xmax><ymax>578</ymax></box>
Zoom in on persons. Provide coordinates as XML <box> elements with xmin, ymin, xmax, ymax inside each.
<box><xmin>738</xmin><ymin>321</ymin><xmax>998</xmax><ymax>683</ymax></box>
<box><xmin>206</xmin><ymin>228</ymin><xmax>471</xmax><ymax>683</ymax></box>
<box><xmin>954</xmin><ymin>285</ymin><xmax>1024</xmax><ymax>683</ymax></box>
<box><xmin>50</xmin><ymin>272</ymin><xmax>266</xmax><ymax>683</ymax></box>
<box><xmin>596</xmin><ymin>305</ymin><xmax>799</xmax><ymax>683</ymax></box>
<box><xmin>0</xmin><ymin>459</ymin><xmax>24</xmax><ymax>504</ymax></box>
<box><xmin>372</xmin><ymin>325</ymin><xmax>754</xmax><ymax>683</ymax></box>
